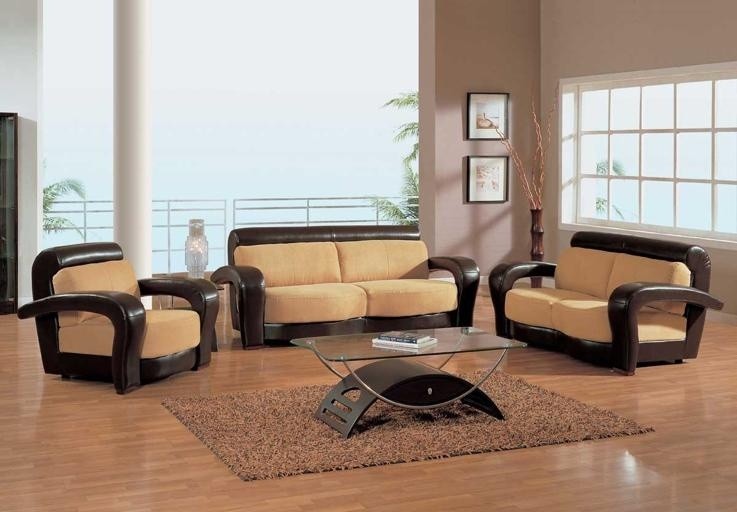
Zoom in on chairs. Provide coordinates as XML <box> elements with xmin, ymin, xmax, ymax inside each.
<box><xmin>15</xmin><ymin>240</ymin><xmax>222</xmax><ymax>397</ymax></box>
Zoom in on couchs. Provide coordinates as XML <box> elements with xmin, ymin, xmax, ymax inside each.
<box><xmin>210</xmin><ymin>225</ymin><xmax>479</xmax><ymax>350</ymax></box>
<box><xmin>487</xmin><ymin>230</ymin><xmax>724</xmax><ymax>377</ymax></box>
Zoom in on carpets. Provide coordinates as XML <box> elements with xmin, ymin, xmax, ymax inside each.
<box><xmin>161</xmin><ymin>367</ymin><xmax>654</xmax><ymax>482</ymax></box>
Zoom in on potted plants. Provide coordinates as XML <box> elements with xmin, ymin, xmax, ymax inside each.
<box><xmin>482</xmin><ymin>75</ymin><xmax>567</xmax><ymax>287</ymax></box>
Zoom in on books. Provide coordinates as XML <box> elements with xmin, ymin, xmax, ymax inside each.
<box><xmin>371</xmin><ymin>336</ymin><xmax>438</xmax><ymax>349</ymax></box>
<box><xmin>377</xmin><ymin>330</ymin><xmax>431</xmax><ymax>344</ymax></box>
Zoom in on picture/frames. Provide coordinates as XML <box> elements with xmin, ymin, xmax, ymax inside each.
<box><xmin>466</xmin><ymin>155</ymin><xmax>509</xmax><ymax>204</ymax></box>
<box><xmin>466</xmin><ymin>92</ymin><xmax>509</xmax><ymax>141</ymax></box>
